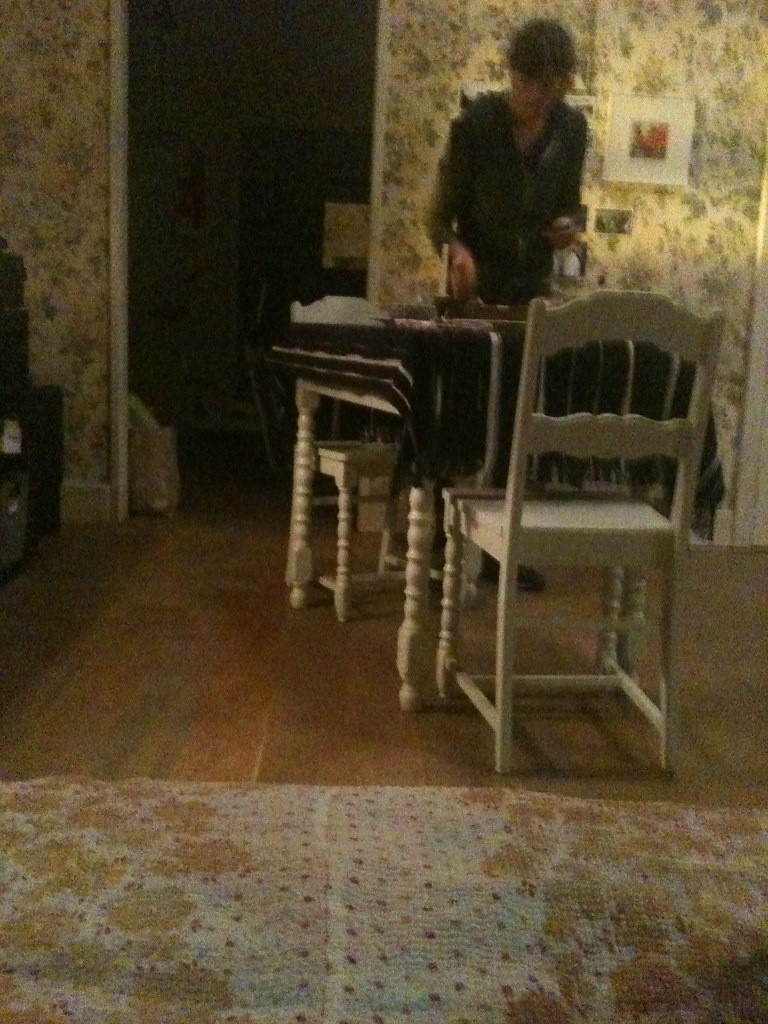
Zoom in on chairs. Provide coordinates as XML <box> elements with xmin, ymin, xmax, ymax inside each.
<box><xmin>292</xmin><ymin>295</ymin><xmax>444</xmax><ymax>620</ymax></box>
<box><xmin>435</xmin><ymin>287</ymin><xmax>726</xmax><ymax>774</ymax></box>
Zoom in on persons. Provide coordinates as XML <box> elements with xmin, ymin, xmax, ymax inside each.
<box><xmin>419</xmin><ymin>19</ymin><xmax>590</xmax><ymax>611</ymax></box>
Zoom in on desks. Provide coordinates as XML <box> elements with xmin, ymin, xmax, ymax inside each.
<box><xmin>268</xmin><ymin>319</ymin><xmax>726</xmax><ymax>714</ymax></box>
